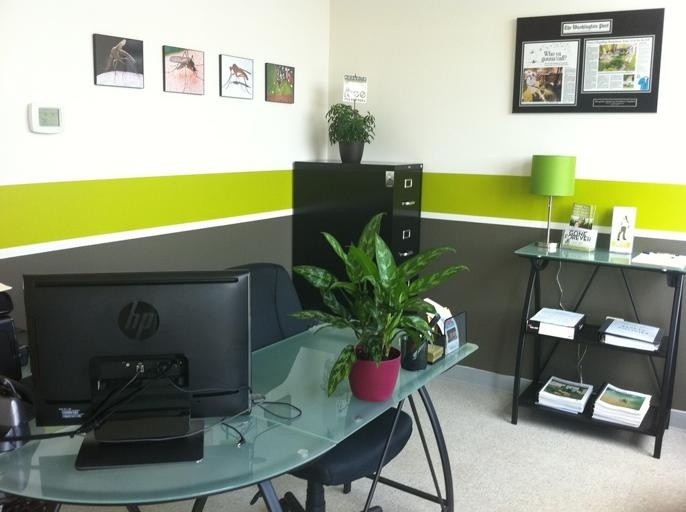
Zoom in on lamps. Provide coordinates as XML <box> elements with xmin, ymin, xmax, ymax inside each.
<box><xmin>528</xmin><ymin>155</ymin><xmax>577</xmax><ymax>247</ymax></box>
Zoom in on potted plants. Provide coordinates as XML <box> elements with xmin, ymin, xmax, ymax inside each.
<box><xmin>286</xmin><ymin>210</ymin><xmax>471</xmax><ymax>403</ymax></box>
<box><xmin>326</xmin><ymin>102</ymin><xmax>379</xmax><ymax>164</ymax></box>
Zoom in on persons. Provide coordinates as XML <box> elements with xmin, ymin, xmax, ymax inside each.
<box><xmin>617</xmin><ymin>215</ymin><xmax>631</xmax><ymax>243</ymax></box>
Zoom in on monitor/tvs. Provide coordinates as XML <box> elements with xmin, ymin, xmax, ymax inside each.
<box><xmin>21</xmin><ymin>271</ymin><xmax>253</xmax><ymax>471</ymax></box>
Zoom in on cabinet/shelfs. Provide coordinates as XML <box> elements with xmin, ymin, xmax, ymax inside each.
<box><xmin>512</xmin><ymin>241</ymin><xmax>684</xmax><ymax>460</ymax></box>
<box><xmin>292</xmin><ymin>161</ymin><xmax>422</xmax><ymax>307</ymax></box>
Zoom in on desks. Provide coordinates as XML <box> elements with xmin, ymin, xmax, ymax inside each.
<box><xmin>1</xmin><ymin>316</ymin><xmax>480</xmax><ymax>512</ymax></box>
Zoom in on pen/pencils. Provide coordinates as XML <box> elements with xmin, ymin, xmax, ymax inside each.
<box><xmin>570</xmin><ymin>214</ymin><xmax>594</xmax><ymax>230</ymax></box>
<box><xmin>411</xmin><ymin>313</ymin><xmax>441</xmax><ymax>360</ymax></box>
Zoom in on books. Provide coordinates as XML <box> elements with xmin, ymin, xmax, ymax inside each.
<box><xmin>568</xmin><ymin>201</ymin><xmax>596</xmax><ymax>230</ymax></box>
<box><xmin>595</xmin><ymin>316</ymin><xmax>664</xmax><ymax>354</ymax></box>
<box><xmin>536</xmin><ymin>313</ymin><xmax>587</xmax><ymax>340</ymax></box>
<box><xmin>537</xmin><ymin>375</ymin><xmax>653</xmax><ymax>429</ymax></box>
<box><xmin>609</xmin><ymin>206</ymin><xmax>637</xmax><ymax>253</ymax></box>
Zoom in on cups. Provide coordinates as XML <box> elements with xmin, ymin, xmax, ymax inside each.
<box><xmin>399</xmin><ymin>333</ymin><xmax>428</xmax><ymax>371</ymax></box>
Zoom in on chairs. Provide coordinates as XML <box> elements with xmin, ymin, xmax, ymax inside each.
<box><xmin>221</xmin><ymin>262</ymin><xmax>412</xmax><ymax>512</ymax></box>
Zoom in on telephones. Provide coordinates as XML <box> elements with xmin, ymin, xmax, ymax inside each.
<box><xmin>0</xmin><ymin>375</ymin><xmax>36</xmax><ymax>452</ymax></box>
